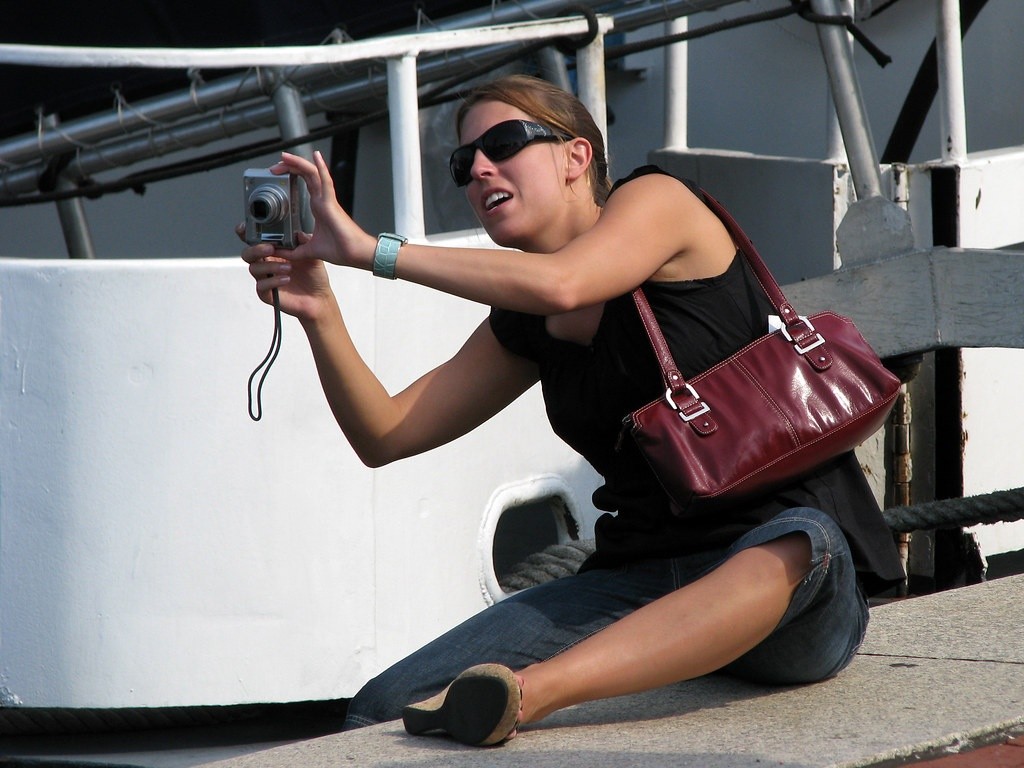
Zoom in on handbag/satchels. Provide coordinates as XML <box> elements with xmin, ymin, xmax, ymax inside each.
<box><xmin>616</xmin><ymin>176</ymin><xmax>903</xmax><ymax>520</ymax></box>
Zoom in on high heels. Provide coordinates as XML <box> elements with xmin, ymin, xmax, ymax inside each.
<box><xmin>403</xmin><ymin>663</ymin><xmax>523</xmax><ymax>747</ymax></box>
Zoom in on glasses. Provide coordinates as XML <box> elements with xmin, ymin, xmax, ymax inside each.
<box><xmin>449</xmin><ymin>119</ymin><xmax>572</xmax><ymax>189</ymax></box>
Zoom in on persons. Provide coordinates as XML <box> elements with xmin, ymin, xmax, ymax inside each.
<box><xmin>237</xmin><ymin>78</ymin><xmax>907</xmax><ymax>746</ymax></box>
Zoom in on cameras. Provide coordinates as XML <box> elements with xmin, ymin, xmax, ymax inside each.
<box><xmin>243</xmin><ymin>168</ymin><xmax>300</xmax><ymax>250</ymax></box>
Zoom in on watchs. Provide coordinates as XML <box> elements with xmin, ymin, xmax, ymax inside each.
<box><xmin>373</xmin><ymin>232</ymin><xmax>408</xmax><ymax>280</ymax></box>
